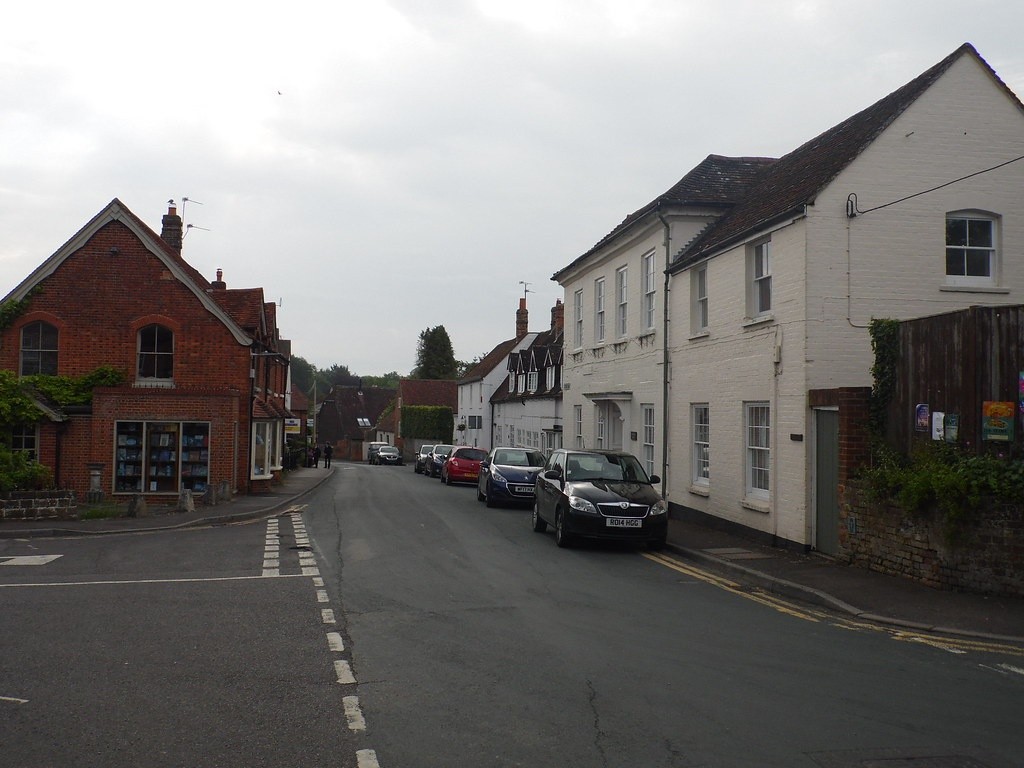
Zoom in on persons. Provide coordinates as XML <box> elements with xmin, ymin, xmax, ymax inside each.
<box><xmin>324</xmin><ymin>441</ymin><xmax>332</xmax><ymax>469</ymax></box>
<box><xmin>312</xmin><ymin>443</ymin><xmax>321</xmax><ymax>468</ymax></box>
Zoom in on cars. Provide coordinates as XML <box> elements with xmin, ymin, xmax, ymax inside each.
<box><xmin>423</xmin><ymin>443</ymin><xmax>455</xmax><ymax>477</ymax></box>
<box><xmin>477</xmin><ymin>446</ymin><xmax>547</xmax><ymax>508</ymax></box>
<box><xmin>373</xmin><ymin>445</ymin><xmax>402</xmax><ymax>466</ymax></box>
<box><xmin>440</xmin><ymin>445</ymin><xmax>489</xmax><ymax>486</ymax></box>
<box><xmin>413</xmin><ymin>444</ymin><xmax>433</xmax><ymax>474</ymax></box>
<box><xmin>531</xmin><ymin>448</ymin><xmax>669</xmax><ymax>547</ymax></box>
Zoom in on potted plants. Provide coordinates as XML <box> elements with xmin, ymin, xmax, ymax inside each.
<box><xmin>457</xmin><ymin>424</ymin><xmax>466</xmax><ymax>432</ymax></box>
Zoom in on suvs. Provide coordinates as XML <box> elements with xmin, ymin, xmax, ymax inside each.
<box><xmin>367</xmin><ymin>442</ymin><xmax>389</xmax><ymax>464</ymax></box>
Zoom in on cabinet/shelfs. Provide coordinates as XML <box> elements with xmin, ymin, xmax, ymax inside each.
<box><xmin>112</xmin><ymin>420</ymin><xmax>211</xmax><ymax>495</ymax></box>
